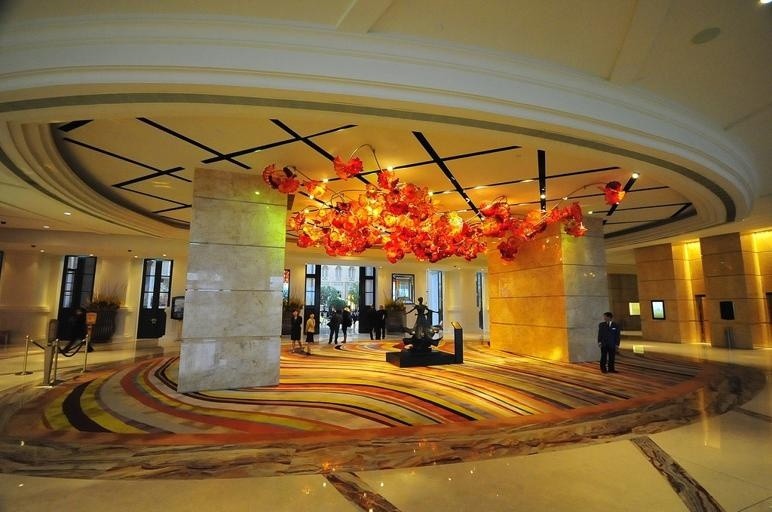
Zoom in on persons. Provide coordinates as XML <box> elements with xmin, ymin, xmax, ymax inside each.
<box><xmin>62</xmin><ymin>307</ymin><xmax>94</xmax><ymax>352</ymax></box>
<box><xmin>405</xmin><ymin>297</ymin><xmax>435</xmax><ymax>338</ymax></box>
<box><xmin>321</xmin><ymin>305</ymin><xmax>387</xmax><ymax>344</ymax></box>
<box><xmin>289</xmin><ymin>308</ymin><xmax>303</xmax><ymax>353</ymax></box>
<box><xmin>305</xmin><ymin>312</ymin><xmax>316</xmax><ymax>354</ymax></box>
<box><xmin>598</xmin><ymin>312</ymin><xmax>620</xmax><ymax>373</ymax></box>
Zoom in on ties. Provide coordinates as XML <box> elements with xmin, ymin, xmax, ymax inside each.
<box><xmin>607</xmin><ymin>321</ymin><xmax>611</xmax><ymax>328</ymax></box>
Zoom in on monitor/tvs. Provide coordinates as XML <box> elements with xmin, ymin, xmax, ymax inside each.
<box><xmin>170</xmin><ymin>296</ymin><xmax>184</xmax><ymax>321</ymax></box>
